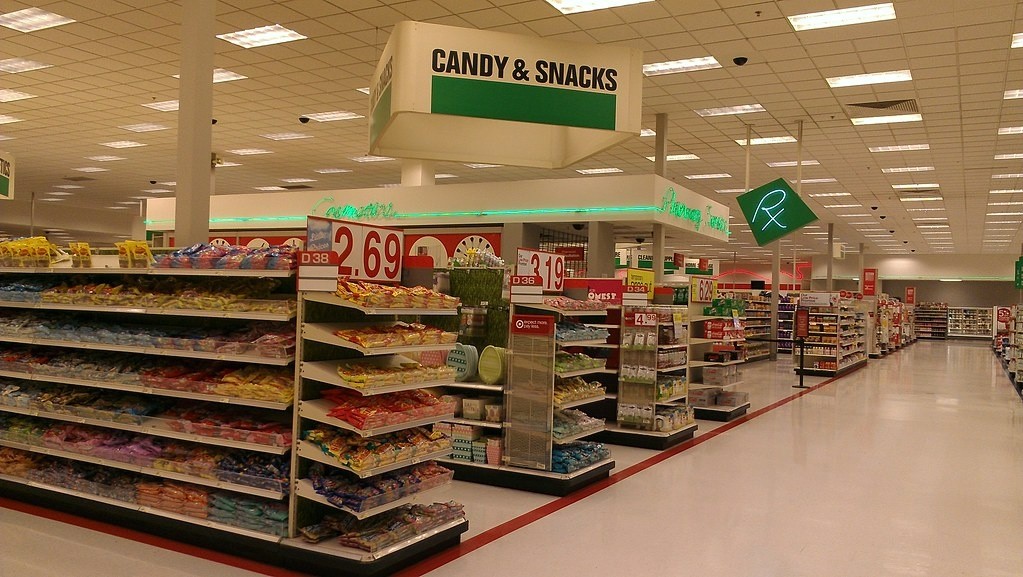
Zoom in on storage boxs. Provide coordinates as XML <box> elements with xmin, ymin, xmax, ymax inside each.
<box><xmin>716</xmin><ymin>392</ymin><xmax>749</xmax><ymax>407</ymax></box>
<box><xmin>484</xmin><ymin>404</ymin><xmax>504</xmax><ymax>422</ymax></box>
<box><xmin>703</xmin><ymin>367</ymin><xmax>725</xmax><ymax>386</ymax></box>
<box><xmin>689</xmin><ymin>389</ymin><xmax>715</xmax><ymax>407</ymax></box>
<box><xmin>463</xmin><ymin>397</ymin><xmax>494</xmax><ymax>419</ymax></box>
<box><xmin>726</xmin><ymin>365</ymin><xmax>736</xmax><ymax>385</ymax></box>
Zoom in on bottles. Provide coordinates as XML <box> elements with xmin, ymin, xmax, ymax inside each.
<box><xmin>795</xmin><ymin>345</ymin><xmax>836</xmax><ymax>355</ymax></box>
<box><xmin>778</xmin><ymin>342</ymin><xmax>791</xmax><ymax>348</ymax></box>
<box><xmin>778</xmin><ymin>322</ymin><xmax>792</xmax><ymax>329</ymax></box>
<box><xmin>813</xmin><ymin>361</ymin><xmax>836</xmax><ymax>369</ymax></box>
<box><xmin>780</xmin><ymin>296</ymin><xmax>794</xmax><ymax>303</ymax></box>
<box><xmin>779</xmin><ymin>332</ymin><xmax>790</xmax><ymax>338</ymax></box>
<box><xmin>779</xmin><ymin>305</ymin><xmax>795</xmax><ymax>310</ymax></box>
<box><xmin>779</xmin><ymin>313</ymin><xmax>793</xmax><ymax>320</ymax></box>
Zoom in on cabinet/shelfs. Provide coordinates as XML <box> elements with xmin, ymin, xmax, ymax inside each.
<box><xmin>778</xmin><ymin>290</ymin><xmax>882</xmax><ymax>358</ymax></box>
<box><xmin>888</xmin><ymin>300</ymin><xmax>895</xmax><ymax>352</ymax></box>
<box><xmin>880</xmin><ymin>293</ymin><xmax>890</xmax><ymax>354</ymax></box>
<box><xmin>560</xmin><ymin>267</ymin><xmax>698</xmax><ymax>451</ymax></box>
<box><xmin>720</xmin><ymin>291</ymin><xmax>771</xmax><ymax>364</ymax></box>
<box><xmin>915</xmin><ymin>302</ymin><xmax>1023</xmax><ymax>389</ymax></box>
<box><xmin>792</xmin><ymin>291</ymin><xmax>869</xmax><ymax>378</ymax></box>
<box><xmin>420</xmin><ymin>248</ymin><xmax>615</xmax><ymax>497</ymax></box>
<box><xmin>896</xmin><ymin>302</ymin><xmax>915</xmax><ymax>349</ymax></box>
<box><xmin>650</xmin><ymin>275</ymin><xmax>750</xmax><ymax>423</ymax></box>
<box><xmin>0</xmin><ymin>215</ymin><xmax>468</xmax><ymax>577</ymax></box>
